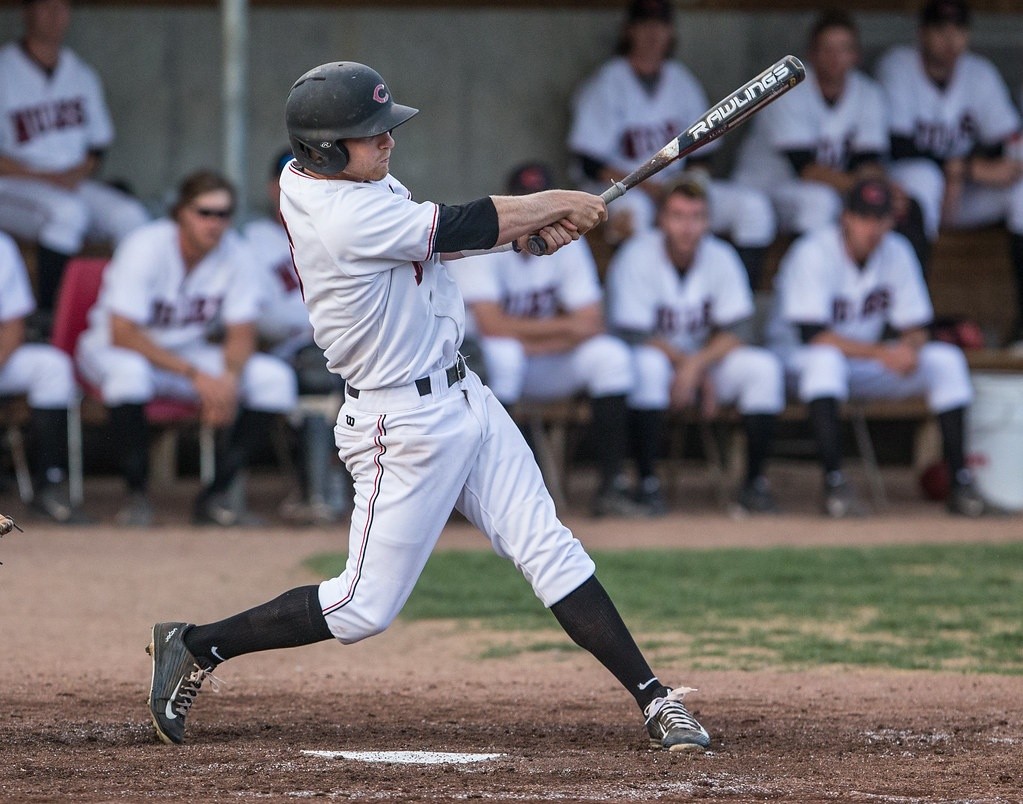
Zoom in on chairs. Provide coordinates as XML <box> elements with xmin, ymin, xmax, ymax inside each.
<box><xmin>1</xmin><ymin>262</ymin><xmax>933</xmax><ymax>518</ymax></box>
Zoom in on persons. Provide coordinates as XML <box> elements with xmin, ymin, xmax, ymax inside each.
<box><xmin>0</xmin><ymin>0</ymin><xmax>1022</xmax><ymax>518</ymax></box>
<box><xmin>147</xmin><ymin>60</ymin><xmax>712</xmax><ymax>755</ymax></box>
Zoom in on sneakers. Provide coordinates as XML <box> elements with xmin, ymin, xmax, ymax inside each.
<box><xmin>642</xmin><ymin>686</ymin><xmax>711</xmax><ymax>753</ymax></box>
<box><xmin>148</xmin><ymin>622</ymin><xmax>227</xmax><ymax>745</ymax></box>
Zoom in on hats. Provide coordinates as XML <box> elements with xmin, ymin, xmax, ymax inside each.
<box><xmin>847</xmin><ymin>175</ymin><xmax>891</xmax><ymax>219</ymax></box>
<box><xmin>507</xmin><ymin>162</ymin><xmax>560</xmax><ymax>193</ymax></box>
<box><xmin>660</xmin><ymin>171</ymin><xmax>715</xmax><ymax>204</ymax></box>
<box><xmin>920</xmin><ymin>0</ymin><xmax>975</xmax><ymax>30</ymax></box>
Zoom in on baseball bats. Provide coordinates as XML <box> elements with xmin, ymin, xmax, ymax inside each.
<box><xmin>526</xmin><ymin>53</ymin><xmax>808</xmax><ymax>256</ymax></box>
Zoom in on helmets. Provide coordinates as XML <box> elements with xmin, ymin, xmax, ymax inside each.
<box><xmin>285</xmin><ymin>61</ymin><xmax>419</xmax><ymax>176</ymax></box>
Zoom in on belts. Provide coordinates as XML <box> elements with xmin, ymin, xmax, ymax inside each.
<box><xmin>347</xmin><ymin>357</ymin><xmax>467</xmax><ymax>399</ymax></box>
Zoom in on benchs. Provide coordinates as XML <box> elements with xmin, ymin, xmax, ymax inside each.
<box><xmin>579</xmin><ymin>215</ymin><xmax>1023</xmax><ymax>359</ymax></box>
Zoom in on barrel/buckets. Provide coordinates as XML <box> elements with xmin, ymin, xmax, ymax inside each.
<box><xmin>964</xmin><ymin>376</ymin><xmax>1023</xmax><ymax>514</ymax></box>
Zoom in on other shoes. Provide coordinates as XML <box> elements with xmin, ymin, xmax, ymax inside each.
<box><xmin>30</xmin><ymin>486</ymin><xmax>76</xmax><ymax>524</ymax></box>
<box><xmin>946</xmin><ymin>462</ymin><xmax>984</xmax><ymax>517</ymax></box>
<box><xmin>120</xmin><ymin>490</ymin><xmax>153</xmax><ymax>524</ymax></box>
<box><xmin>630</xmin><ymin>482</ymin><xmax>670</xmax><ymax>512</ymax></box>
<box><xmin>731</xmin><ymin>485</ymin><xmax>780</xmax><ymax>518</ymax></box>
<box><xmin>204</xmin><ymin>484</ymin><xmax>241</xmax><ymax>527</ymax></box>
<box><xmin>821</xmin><ymin>468</ymin><xmax>853</xmax><ymax>518</ymax></box>
<box><xmin>595</xmin><ymin>484</ymin><xmax>636</xmax><ymax>520</ymax></box>
<box><xmin>283</xmin><ymin>487</ymin><xmax>334</xmax><ymax>525</ymax></box>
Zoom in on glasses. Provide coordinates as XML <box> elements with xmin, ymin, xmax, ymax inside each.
<box><xmin>188</xmin><ymin>205</ymin><xmax>231</xmax><ymax>219</ymax></box>
